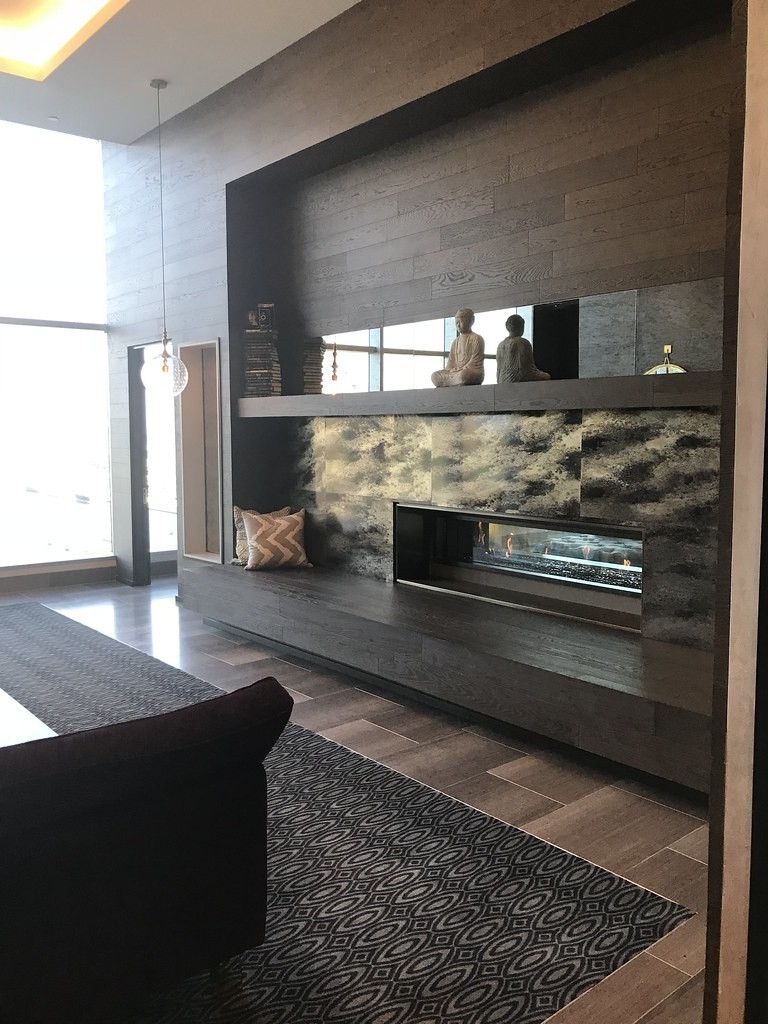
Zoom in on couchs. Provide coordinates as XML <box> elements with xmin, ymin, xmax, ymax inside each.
<box><xmin>0</xmin><ymin>676</ymin><xmax>295</xmax><ymax>1009</ymax></box>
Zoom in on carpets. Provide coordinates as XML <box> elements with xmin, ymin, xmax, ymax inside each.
<box><xmin>0</xmin><ymin>592</ymin><xmax>699</xmax><ymax>1023</ymax></box>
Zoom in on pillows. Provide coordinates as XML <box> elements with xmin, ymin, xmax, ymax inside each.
<box><xmin>241</xmin><ymin>508</ymin><xmax>313</xmax><ymax>570</ymax></box>
<box><xmin>228</xmin><ymin>505</ymin><xmax>293</xmax><ymax>569</ymax></box>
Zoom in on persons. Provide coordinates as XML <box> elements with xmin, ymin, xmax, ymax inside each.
<box><xmin>496</xmin><ymin>315</ymin><xmax>550</xmax><ymax>383</ymax></box>
<box><xmin>431</xmin><ymin>308</ymin><xmax>485</xmax><ymax>388</ymax></box>
